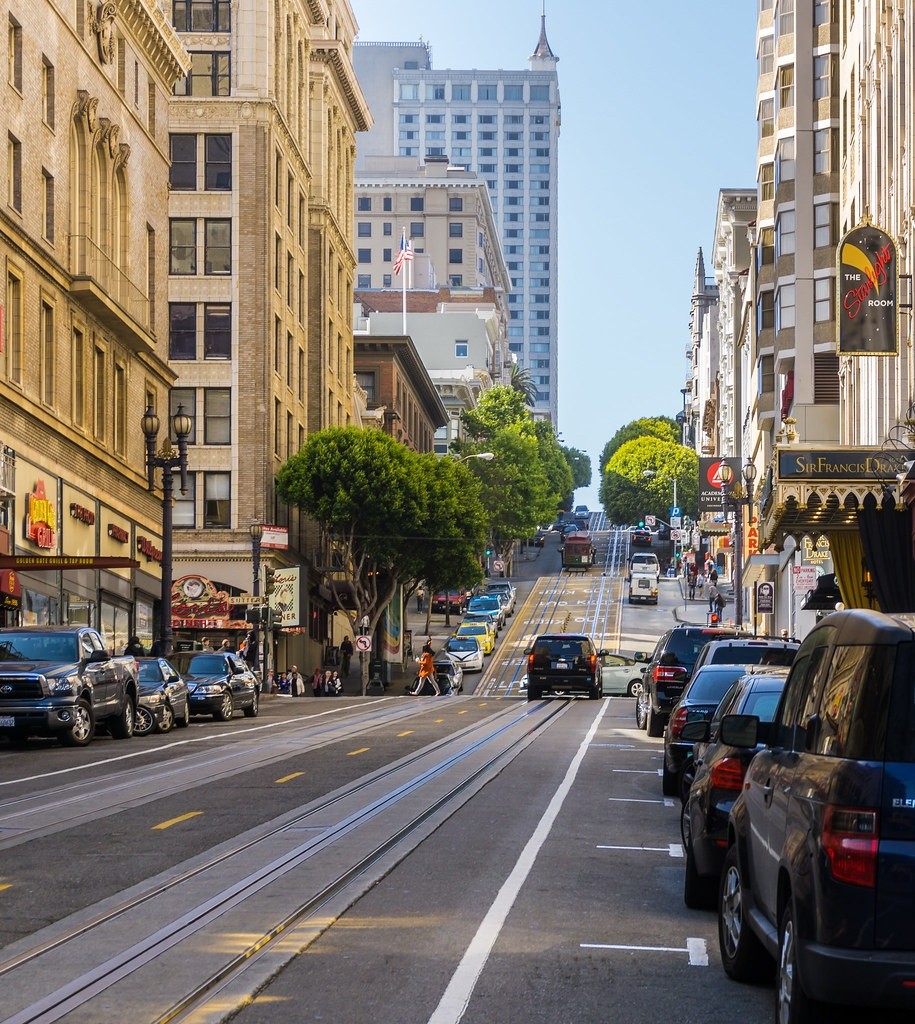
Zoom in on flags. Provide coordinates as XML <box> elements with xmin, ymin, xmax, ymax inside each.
<box><xmin>393</xmin><ymin>233</ymin><xmax>413</xmax><ymax>275</ymax></box>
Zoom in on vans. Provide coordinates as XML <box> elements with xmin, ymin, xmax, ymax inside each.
<box><xmin>626</xmin><ymin>552</ymin><xmax>661</xmax><ymax>583</ymax></box>
<box><xmin>628</xmin><ymin>569</ymin><xmax>660</xmax><ymax>605</ymax></box>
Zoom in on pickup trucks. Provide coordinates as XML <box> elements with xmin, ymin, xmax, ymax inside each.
<box><xmin>0</xmin><ymin>626</ymin><xmax>141</xmax><ymax>750</ymax></box>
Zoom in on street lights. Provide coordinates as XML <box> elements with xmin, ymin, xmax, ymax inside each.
<box><xmin>139</xmin><ymin>399</ymin><xmax>192</xmax><ymax>654</ymax></box>
<box><xmin>643</xmin><ymin>470</ymin><xmax>678</xmax><ymax>571</ymax></box>
<box><xmin>249</xmin><ymin>516</ymin><xmax>263</xmax><ymax>672</ymax></box>
<box><xmin>717</xmin><ymin>454</ymin><xmax>759</xmax><ymax>630</ymax></box>
<box><xmin>398</xmin><ymin>451</ymin><xmax>495</xmax><ymax>670</ymax></box>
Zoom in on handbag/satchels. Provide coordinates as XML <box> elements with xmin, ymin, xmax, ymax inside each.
<box><xmin>698</xmin><ymin>584</ymin><xmax>702</xmax><ymax>588</ymax></box>
<box><xmin>722</xmin><ymin>600</ymin><xmax>726</xmax><ymax>607</ymax></box>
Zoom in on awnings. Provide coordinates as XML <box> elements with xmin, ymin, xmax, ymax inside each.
<box><xmin>0</xmin><ymin>569</ymin><xmax>23</xmax><ymax>610</ymax></box>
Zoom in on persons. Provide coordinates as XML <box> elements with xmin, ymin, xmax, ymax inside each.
<box><xmin>708</xmin><ymin>583</ymin><xmax>719</xmax><ymax>613</ymax></box>
<box><xmin>218</xmin><ymin>639</ymin><xmax>231</xmax><ymax>652</ymax></box>
<box><xmin>715</xmin><ymin>594</ymin><xmax>725</xmax><ymax>623</ymax></box>
<box><xmin>416</xmin><ymin>586</ymin><xmax>424</xmax><ymax>615</ymax></box>
<box><xmin>266</xmin><ymin>665</ymin><xmax>305</xmax><ymax>698</ymax></box>
<box><xmin>124</xmin><ymin>636</ymin><xmax>142</xmax><ymax>657</ymax></box>
<box><xmin>410</xmin><ymin>645</ymin><xmax>440</xmax><ymax>696</ymax></box>
<box><xmin>485</xmin><ymin>567</ymin><xmax>491</xmax><ymax>578</ymax></box>
<box><xmin>341</xmin><ymin>636</ymin><xmax>354</xmax><ymax>676</ymax></box>
<box><xmin>688</xmin><ymin>558</ymin><xmax>718</xmax><ymax>600</ymax></box>
<box><xmin>201</xmin><ymin>637</ymin><xmax>210</xmax><ymax>650</ymax></box>
<box><xmin>306</xmin><ymin>668</ymin><xmax>343</xmax><ymax>697</ymax></box>
<box><xmin>781</xmin><ymin>629</ymin><xmax>788</xmax><ymax>642</ymax></box>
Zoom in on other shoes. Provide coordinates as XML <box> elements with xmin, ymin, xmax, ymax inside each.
<box><xmin>708</xmin><ymin>610</ymin><xmax>712</xmax><ymax>612</ymax></box>
<box><xmin>435</xmin><ymin>692</ymin><xmax>441</xmax><ymax>696</ymax></box>
<box><xmin>410</xmin><ymin>691</ymin><xmax>417</xmax><ymax>696</ymax></box>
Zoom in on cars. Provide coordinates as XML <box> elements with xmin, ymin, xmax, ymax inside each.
<box><xmin>441</xmin><ymin>635</ymin><xmax>485</xmax><ymax>673</ymax></box>
<box><xmin>660</xmin><ymin>663</ymin><xmax>790</xmax><ymax>799</ymax></box>
<box><xmin>524</xmin><ymin>520</ymin><xmax>589</xmax><ymax>548</ymax></box>
<box><xmin>404</xmin><ymin>673</ymin><xmax>460</xmax><ymax>697</ymax></box>
<box><xmin>432</xmin><ymin>659</ymin><xmax>464</xmax><ymax>691</ymax></box>
<box><xmin>678</xmin><ymin>674</ymin><xmax>791</xmax><ymax>911</ymax></box>
<box><xmin>524</xmin><ymin>633</ymin><xmax>609</xmax><ymax>700</ymax></box>
<box><xmin>456</xmin><ymin>581</ymin><xmax>517</xmax><ymax>639</ymax></box>
<box><xmin>452</xmin><ymin>621</ymin><xmax>496</xmax><ymax>655</ymax></box>
<box><xmin>518</xmin><ymin>649</ymin><xmax>650</xmax><ymax>698</ymax></box>
<box><xmin>574</xmin><ymin>504</ymin><xmax>590</xmax><ymax>519</ymax></box>
<box><xmin>160</xmin><ymin>650</ymin><xmax>261</xmax><ymax>721</ymax></box>
<box><xmin>432</xmin><ymin>585</ymin><xmax>468</xmax><ymax>615</ymax></box>
<box><xmin>130</xmin><ymin>656</ymin><xmax>190</xmax><ymax>736</ymax></box>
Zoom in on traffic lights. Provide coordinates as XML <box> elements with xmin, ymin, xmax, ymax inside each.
<box><xmin>244</xmin><ymin>608</ymin><xmax>256</xmax><ymax>623</ymax></box>
<box><xmin>267</xmin><ymin>607</ymin><xmax>283</xmax><ymax>631</ymax></box>
<box><xmin>676</xmin><ymin>548</ymin><xmax>681</xmax><ymax>558</ymax></box>
<box><xmin>638</xmin><ymin>511</ymin><xmax>645</xmax><ymax>529</ymax></box>
<box><xmin>264</xmin><ymin>564</ymin><xmax>275</xmax><ymax>597</ymax></box>
<box><xmin>485</xmin><ymin>545</ymin><xmax>492</xmax><ymax>556</ymax></box>
<box><xmin>711</xmin><ymin>615</ymin><xmax>718</xmax><ymax>628</ymax></box>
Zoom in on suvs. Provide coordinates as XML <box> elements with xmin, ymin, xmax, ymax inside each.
<box><xmin>669</xmin><ymin>634</ymin><xmax>801</xmax><ymax>688</ymax></box>
<box><xmin>718</xmin><ymin>607</ymin><xmax>915</xmax><ymax>1024</ymax></box>
<box><xmin>631</xmin><ymin>623</ymin><xmax>753</xmax><ymax>738</ymax></box>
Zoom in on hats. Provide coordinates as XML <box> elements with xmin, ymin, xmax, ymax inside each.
<box><xmin>290</xmin><ymin>665</ymin><xmax>297</xmax><ymax>669</ymax></box>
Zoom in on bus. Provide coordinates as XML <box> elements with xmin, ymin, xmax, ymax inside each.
<box><xmin>557</xmin><ymin>529</ymin><xmax>597</xmax><ymax>573</ymax></box>
